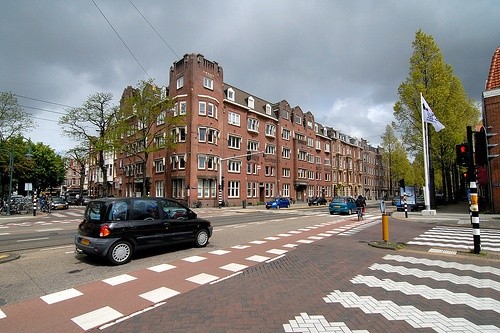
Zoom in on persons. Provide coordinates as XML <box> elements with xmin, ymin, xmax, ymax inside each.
<box><xmin>356</xmin><ymin>195</ymin><xmax>367</xmax><ymax>215</ymax></box>
<box><xmin>39</xmin><ymin>194</ymin><xmax>54</xmax><ymax>213</ymax></box>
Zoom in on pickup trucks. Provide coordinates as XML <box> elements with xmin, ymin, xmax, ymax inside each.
<box><xmin>392</xmin><ymin>186</ymin><xmax>416</xmax><ymax>212</ymax></box>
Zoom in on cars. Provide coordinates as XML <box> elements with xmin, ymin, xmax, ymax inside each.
<box><xmin>74</xmin><ymin>196</ymin><xmax>213</xmax><ymax>266</ymax></box>
<box><xmin>265</xmin><ymin>197</ymin><xmax>290</xmax><ymax>209</ymax></box>
<box><xmin>3</xmin><ymin>189</ymin><xmax>93</xmax><ymax>216</ymax></box>
<box><xmin>308</xmin><ymin>197</ymin><xmax>327</xmax><ymax>206</ymax></box>
<box><xmin>328</xmin><ymin>196</ymin><xmax>359</xmax><ymax>215</ymax></box>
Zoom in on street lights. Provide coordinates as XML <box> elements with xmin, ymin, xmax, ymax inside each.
<box><xmin>218</xmin><ymin>150</ymin><xmax>265</xmax><ymax>206</ymax></box>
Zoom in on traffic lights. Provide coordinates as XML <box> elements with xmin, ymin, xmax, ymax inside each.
<box><xmin>480</xmin><ymin>127</ymin><xmax>499</xmax><ymax>164</ymax></box>
<box><xmin>456</xmin><ymin>144</ymin><xmax>469</xmax><ymax>158</ymax></box>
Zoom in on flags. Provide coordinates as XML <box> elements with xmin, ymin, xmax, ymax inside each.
<box><xmin>423</xmin><ymin>96</ymin><xmax>445</xmax><ymax>132</ymax></box>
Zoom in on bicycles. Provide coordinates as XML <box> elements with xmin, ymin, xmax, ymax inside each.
<box><xmin>357</xmin><ymin>207</ymin><xmax>364</xmax><ymax>221</ymax></box>
